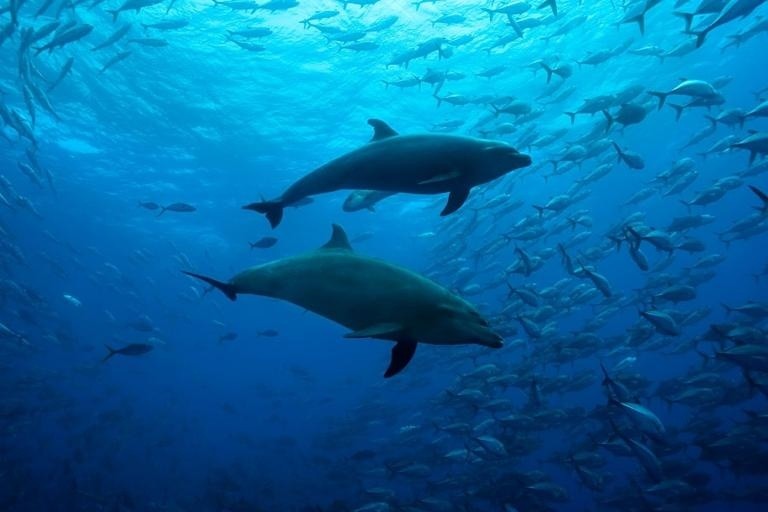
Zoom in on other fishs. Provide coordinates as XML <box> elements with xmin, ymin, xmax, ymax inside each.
<box><xmin>0</xmin><ymin>0</ymin><xmax>768</xmax><ymax>512</ymax></box>
